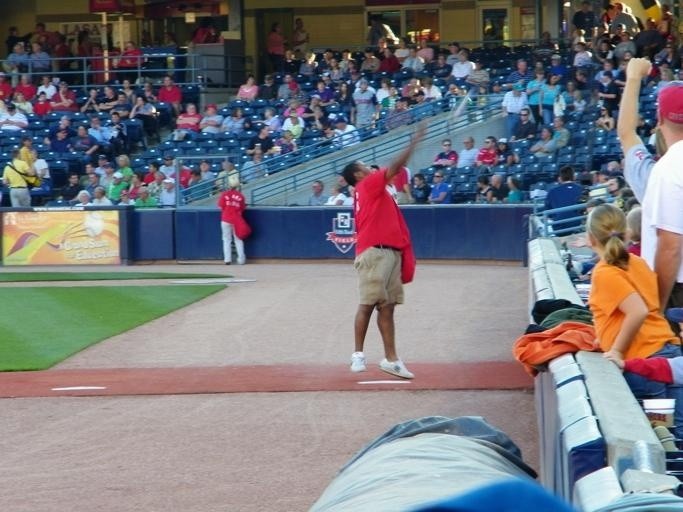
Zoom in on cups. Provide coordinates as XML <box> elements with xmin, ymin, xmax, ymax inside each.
<box><xmin>642</xmin><ymin>398</ymin><xmax>678</xmax><ymax>427</ymax></box>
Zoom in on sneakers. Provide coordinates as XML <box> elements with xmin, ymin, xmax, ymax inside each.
<box><xmin>351</xmin><ymin>352</ymin><xmax>368</xmax><ymax>372</ymax></box>
<box><xmin>225</xmin><ymin>260</ymin><xmax>232</xmax><ymax>265</ymax></box>
<box><xmin>378</xmin><ymin>358</ymin><xmax>415</xmax><ymax>379</ymax></box>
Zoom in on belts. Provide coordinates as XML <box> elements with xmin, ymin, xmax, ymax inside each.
<box><xmin>374</xmin><ymin>244</ymin><xmax>400</xmax><ymax>251</ymax></box>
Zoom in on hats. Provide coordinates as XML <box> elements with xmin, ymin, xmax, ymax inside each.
<box><xmin>658</xmin><ymin>80</ymin><xmax>683</xmax><ymax>124</ymax></box>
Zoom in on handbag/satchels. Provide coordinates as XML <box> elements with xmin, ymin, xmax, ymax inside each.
<box><xmin>234</xmin><ymin>215</ymin><xmax>252</xmax><ymax>239</ymax></box>
<box><xmin>401</xmin><ymin>226</ymin><xmax>416</xmax><ymax>284</ymax></box>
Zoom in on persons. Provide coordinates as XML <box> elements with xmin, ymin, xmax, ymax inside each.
<box><xmin>216</xmin><ymin>173</ymin><xmax>249</xmax><ymax>267</ymax></box>
<box><xmin>0</xmin><ymin>1</ymin><xmax>682</xmax><ymax>208</ymax></box>
<box><xmin>597</xmin><ymin>303</ymin><xmax>683</xmax><ymax>389</ymax></box>
<box><xmin>584</xmin><ymin>202</ymin><xmax>682</xmax><ymax>453</ymax></box>
<box><xmin>336</xmin><ymin>114</ymin><xmax>430</xmax><ymax>381</ymax></box>
<box><xmin>637</xmin><ymin>82</ymin><xmax>682</xmax><ymax>344</ymax></box>
<box><xmin>617</xmin><ymin>203</ymin><xmax>641</xmax><ymax>259</ymax></box>
